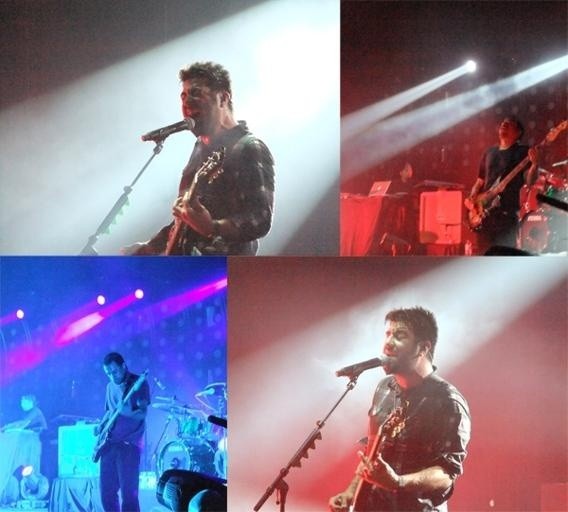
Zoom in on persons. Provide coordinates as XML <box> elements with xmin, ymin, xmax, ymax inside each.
<box><xmin>328</xmin><ymin>307</ymin><xmax>473</xmax><ymax>511</ymax></box>
<box><xmin>469</xmin><ymin>114</ymin><xmax>543</xmax><ymax>258</ymax></box>
<box><xmin>119</xmin><ymin>61</ymin><xmax>274</xmax><ymax>256</ymax></box>
<box><xmin>94</xmin><ymin>352</ymin><xmax>152</xmax><ymax>510</ymax></box>
<box><xmin>387</xmin><ymin>157</ymin><xmax>419</xmax><ymax>196</ymax></box>
<box><xmin>19</xmin><ymin>393</ymin><xmax>48</xmax><ymax>434</ymax></box>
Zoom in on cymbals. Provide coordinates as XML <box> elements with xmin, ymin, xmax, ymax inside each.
<box><xmin>152</xmin><ymin>402</ymin><xmax>192</xmax><ymax>414</ymax></box>
<box><xmin>204</xmin><ymin>382</ymin><xmax>227</xmax><ymax>405</ymax></box>
<box><xmin>155</xmin><ymin>396</ymin><xmax>192</xmax><ymax>406</ymax></box>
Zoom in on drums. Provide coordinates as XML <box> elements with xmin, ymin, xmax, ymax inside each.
<box><xmin>537</xmin><ymin>177</ymin><xmax>568</xmax><ymax>216</ymax></box>
<box><xmin>158</xmin><ymin>438</ymin><xmax>217</xmax><ymax>478</ymax></box>
<box><xmin>177</xmin><ymin>409</ymin><xmax>211</xmax><ymax>439</ymax></box>
<box><xmin>517</xmin><ymin>208</ymin><xmax>568</xmax><ymax>254</ymax></box>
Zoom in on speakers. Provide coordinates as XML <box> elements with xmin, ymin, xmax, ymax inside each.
<box><xmin>57</xmin><ymin>423</ymin><xmax>100</xmax><ymax>477</ymax></box>
<box><xmin>536</xmin><ymin>479</ymin><xmax>568</xmax><ymax>512</ymax></box>
<box><xmin>418</xmin><ymin>190</ymin><xmax>469</xmax><ymax>245</ymax></box>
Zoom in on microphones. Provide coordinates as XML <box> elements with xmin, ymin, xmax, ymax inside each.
<box><xmin>151</xmin><ymin>375</ymin><xmax>169</xmax><ymax>394</ymax></box>
<box><xmin>335</xmin><ymin>354</ymin><xmax>386</xmax><ymax>377</ymax></box>
<box><xmin>169</xmin><ymin>459</ymin><xmax>178</xmax><ymax>467</ymax></box>
<box><xmin>195</xmin><ymin>388</ymin><xmax>215</xmax><ymax>397</ymax></box>
<box><xmin>141</xmin><ymin>116</ymin><xmax>196</xmax><ymax>142</ymax></box>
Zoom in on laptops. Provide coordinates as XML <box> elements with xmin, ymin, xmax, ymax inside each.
<box><xmin>368</xmin><ymin>180</ymin><xmax>399</xmax><ymax>198</ymax></box>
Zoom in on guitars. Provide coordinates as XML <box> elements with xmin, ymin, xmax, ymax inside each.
<box><xmin>467</xmin><ymin>120</ymin><xmax>568</xmax><ymax>235</ymax></box>
<box><xmin>164</xmin><ymin>145</ymin><xmax>225</xmax><ymax>257</ymax></box>
<box><xmin>92</xmin><ymin>369</ymin><xmax>150</xmax><ymax>462</ymax></box>
<box><xmin>348</xmin><ymin>400</ymin><xmax>410</xmax><ymax>512</ymax></box>
